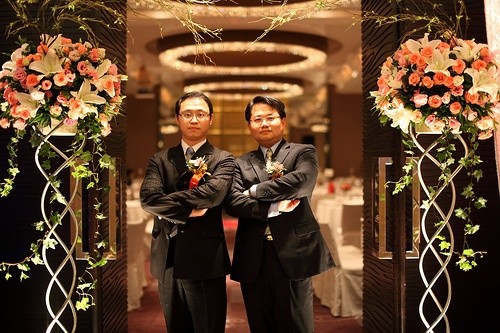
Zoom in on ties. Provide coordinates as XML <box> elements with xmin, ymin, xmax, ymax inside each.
<box><xmin>168</xmin><ymin>147</ymin><xmax>193</xmax><ymax>239</ymax></box>
<box><xmin>264</xmin><ymin>149</ymin><xmax>273</xmax><ymax>235</ymax></box>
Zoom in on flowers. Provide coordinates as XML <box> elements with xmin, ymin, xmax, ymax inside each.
<box><xmin>370</xmin><ymin>35</ymin><xmax>500</xmax><ymax>140</ymax></box>
<box><xmin>266</xmin><ymin>162</ymin><xmax>286</xmax><ymax>180</ymax></box>
<box><xmin>0</xmin><ymin>34</ymin><xmax>129</xmax><ymax>138</ymax></box>
<box><xmin>186</xmin><ymin>155</ymin><xmax>207</xmax><ymax>174</ymax></box>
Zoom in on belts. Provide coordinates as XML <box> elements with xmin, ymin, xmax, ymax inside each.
<box><xmin>263</xmin><ymin>234</ymin><xmax>274</xmax><ymax>241</ymax></box>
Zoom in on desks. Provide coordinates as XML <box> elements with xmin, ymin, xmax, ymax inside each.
<box><xmin>126</xmin><ymin>200</ymin><xmax>154</xmax><ymax>233</ymax></box>
<box><xmin>310</xmin><ymin>178</ymin><xmax>363</xmax><ymax>214</ymax></box>
<box><xmin>317</xmin><ymin>197</ymin><xmax>363</xmax><ymax>236</ymax></box>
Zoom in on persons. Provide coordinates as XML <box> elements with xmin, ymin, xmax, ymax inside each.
<box><xmin>140</xmin><ymin>91</ymin><xmax>236</xmax><ymax>333</ymax></box>
<box><xmin>225</xmin><ymin>96</ymin><xmax>336</xmax><ymax>333</ymax></box>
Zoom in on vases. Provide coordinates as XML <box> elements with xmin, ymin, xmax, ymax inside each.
<box><xmin>415</xmin><ymin>116</ymin><xmax>462</xmax><ymax>134</ymax></box>
<box><xmin>37</xmin><ymin>117</ymin><xmax>77</xmax><ymax>136</ymax></box>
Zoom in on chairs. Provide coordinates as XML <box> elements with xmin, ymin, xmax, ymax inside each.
<box><xmin>126</xmin><ymin>215</ymin><xmax>153</xmax><ymax>311</ymax></box>
<box><xmin>337</xmin><ymin>204</ymin><xmax>364</xmax><ymax>246</ymax></box>
<box><xmin>312</xmin><ymin>222</ymin><xmax>364</xmax><ymax>316</ymax></box>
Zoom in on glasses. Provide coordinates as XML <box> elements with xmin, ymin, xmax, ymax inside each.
<box><xmin>176</xmin><ymin>110</ymin><xmax>211</xmax><ymax>120</ymax></box>
<box><xmin>249</xmin><ymin>114</ymin><xmax>281</xmax><ymax>124</ymax></box>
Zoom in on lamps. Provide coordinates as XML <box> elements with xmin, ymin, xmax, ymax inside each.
<box><xmin>148</xmin><ymin>0</ymin><xmax>350</xmax><ymax>17</ymax></box>
<box><xmin>310</xmin><ymin>117</ymin><xmax>329</xmax><ymax>133</ymax></box>
<box><xmin>158</xmin><ymin>117</ymin><xmax>178</xmax><ymax>134</ymax></box>
<box><xmin>183</xmin><ymin>75</ymin><xmax>304</xmax><ymax>100</ymax></box>
<box><xmin>157</xmin><ymin>28</ymin><xmax>327</xmax><ymax>74</ymax></box>
